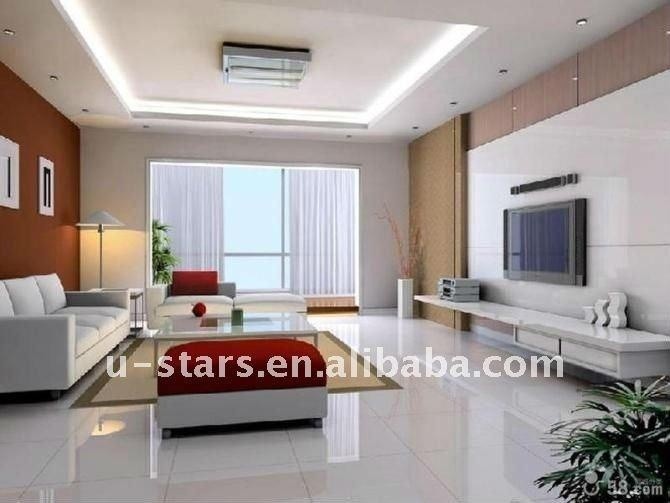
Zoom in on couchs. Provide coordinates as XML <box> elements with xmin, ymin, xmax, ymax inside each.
<box><xmin>0</xmin><ymin>272</ymin><xmax>131</xmax><ymax>402</ymax></box>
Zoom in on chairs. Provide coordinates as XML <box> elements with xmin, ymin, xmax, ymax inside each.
<box><xmin>144</xmin><ymin>271</ymin><xmax>236</xmax><ymax>332</ymax></box>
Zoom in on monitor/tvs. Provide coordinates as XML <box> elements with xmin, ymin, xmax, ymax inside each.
<box><xmin>503</xmin><ymin>198</ymin><xmax>587</xmax><ymax>286</ymax></box>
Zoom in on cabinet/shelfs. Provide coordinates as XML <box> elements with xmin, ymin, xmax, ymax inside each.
<box><xmin>413</xmin><ymin>295</ymin><xmax>670</xmax><ymax>380</ymax></box>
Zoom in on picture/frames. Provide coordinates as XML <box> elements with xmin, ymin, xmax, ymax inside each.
<box><xmin>38</xmin><ymin>155</ymin><xmax>55</xmax><ymax>216</ymax></box>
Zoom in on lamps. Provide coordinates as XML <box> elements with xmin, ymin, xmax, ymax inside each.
<box><xmin>75</xmin><ymin>210</ymin><xmax>125</xmax><ymax>292</ymax></box>
<box><xmin>92</xmin><ymin>419</ymin><xmax>126</xmax><ymax>435</ymax></box>
<box><xmin>221</xmin><ymin>41</ymin><xmax>311</xmax><ymax>88</ymax></box>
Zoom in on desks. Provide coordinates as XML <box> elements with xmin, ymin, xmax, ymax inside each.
<box><xmin>89</xmin><ymin>288</ymin><xmax>144</xmax><ymax>338</ymax></box>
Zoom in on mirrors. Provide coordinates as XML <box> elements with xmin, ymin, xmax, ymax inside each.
<box><xmin>0</xmin><ymin>135</ymin><xmax>19</xmax><ymax>209</ymax></box>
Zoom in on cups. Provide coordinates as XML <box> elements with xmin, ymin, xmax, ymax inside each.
<box><xmin>232</xmin><ymin>309</ymin><xmax>243</xmax><ymax>325</ymax></box>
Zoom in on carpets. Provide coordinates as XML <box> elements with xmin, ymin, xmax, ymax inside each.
<box><xmin>70</xmin><ymin>331</ymin><xmax>403</xmax><ymax>409</ymax></box>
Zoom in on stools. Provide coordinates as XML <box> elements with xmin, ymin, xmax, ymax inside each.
<box><xmin>157</xmin><ymin>338</ymin><xmax>327</xmax><ymax>440</ymax></box>
<box><xmin>234</xmin><ymin>294</ymin><xmax>307</xmax><ymax>320</ymax></box>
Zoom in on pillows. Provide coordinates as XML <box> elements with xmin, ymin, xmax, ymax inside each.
<box><xmin>172</xmin><ymin>271</ymin><xmax>218</xmax><ymax>295</ymax></box>
<box><xmin>35</xmin><ymin>273</ymin><xmax>67</xmax><ymax>313</ymax></box>
<box><xmin>0</xmin><ymin>280</ymin><xmax>14</xmax><ymax>316</ymax></box>
<box><xmin>4</xmin><ymin>276</ymin><xmax>46</xmax><ymax>316</ymax></box>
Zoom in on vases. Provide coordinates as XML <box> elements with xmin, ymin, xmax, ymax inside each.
<box><xmin>397</xmin><ymin>279</ymin><xmax>414</xmax><ymax>319</ymax></box>
